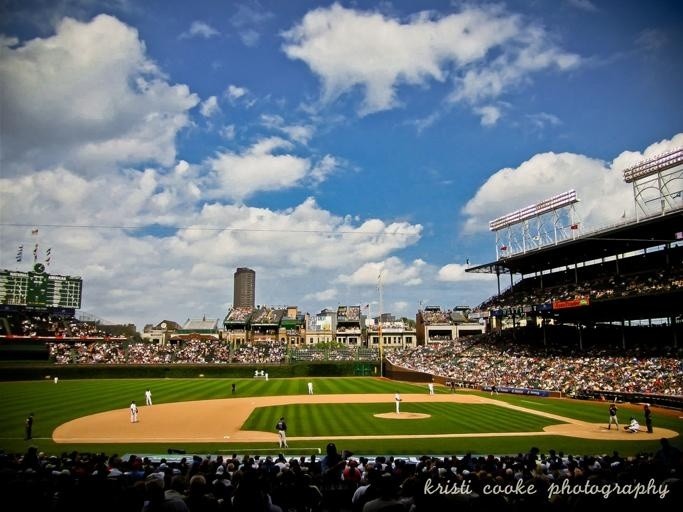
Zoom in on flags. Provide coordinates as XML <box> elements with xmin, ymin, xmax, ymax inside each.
<box><xmin>672</xmin><ymin>191</ymin><xmax>681</xmax><ymax>199</ymax></box>
<box><xmin>570</xmin><ymin>224</ymin><xmax>578</xmax><ymax>230</ymax></box>
<box><xmin>500</xmin><ymin>246</ymin><xmax>506</xmax><ymax>251</ymax></box>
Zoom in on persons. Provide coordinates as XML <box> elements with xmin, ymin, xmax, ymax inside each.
<box><xmin>1</xmin><ymin>437</ymin><xmax>683</xmax><ymax>512</ymax></box>
<box><xmin>265</xmin><ymin>372</ymin><xmax>268</xmax><ymax>382</ymax></box>
<box><xmin>306</xmin><ymin>380</ymin><xmax>313</xmax><ymax>395</ymax></box>
<box><xmin>434</xmin><ymin>271</ymin><xmax>681</xmax><ymax>401</ymax></box>
<box><xmin>427</xmin><ymin>380</ymin><xmax>434</xmax><ymax>395</ymax></box>
<box><xmin>129</xmin><ymin>399</ymin><xmax>137</xmax><ymax>423</ymax></box>
<box><xmin>143</xmin><ymin>385</ymin><xmax>152</xmax><ymax>406</ymax></box>
<box><xmin>275</xmin><ymin>416</ymin><xmax>288</xmax><ymax>448</ymax></box>
<box><xmin>607</xmin><ymin>402</ymin><xmax>619</xmax><ymax>430</ymax></box>
<box><xmin>52</xmin><ymin>376</ymin><xmax>57</xmax><ymax>384</ymax></box>
<box><xmin>230</xmin><ymin>381</ymin><xmax>235</xmax><ymax>394</ymax></box>
<box><xmin>8</xmin><ymin>307</ymin><xmax>433</xmax><ymax>379</ymax></box>
<box><xmin>394</xmin><ymin>389</ymin><xmax>402</xmax><ymax>415</ymax></box>
<box><xmin>643</xmin><ymin>404</ymin><xmax>654</xmax><ymax>433</ymax></box>
<box><xmin>623</xmin><ymin>415</ymin><xmax>640</xmax><ymax>433</ymax></box>
<box><xmin>24</xmin><ymin>412</ymin><xmax>34</xmax><ymax>441</ymax></box>
